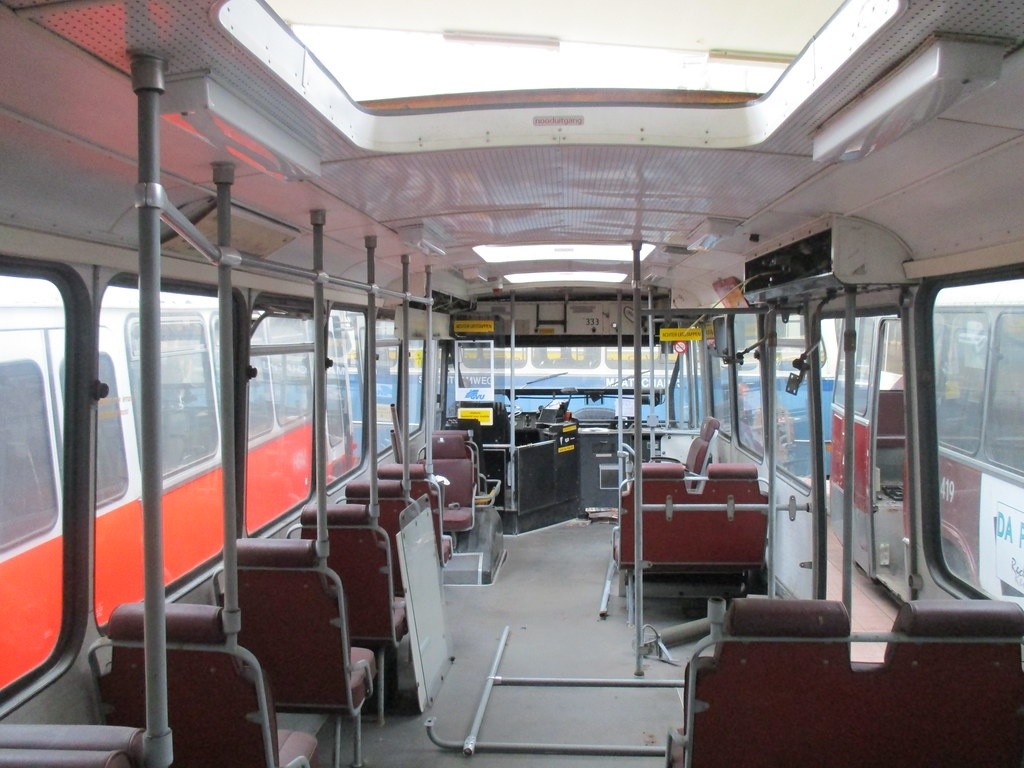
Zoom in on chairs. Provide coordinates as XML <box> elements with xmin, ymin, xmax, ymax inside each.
<box><xmin>0</xmin><ymin>430</ymin><xmax>479</xmax><ymax>768</ymax></box>
<box><xmin>679</xmin><ymin>417</ymin><xmax>722</xmax><ymax>478</ymax></box>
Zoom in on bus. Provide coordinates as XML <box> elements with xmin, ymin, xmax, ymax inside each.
<box><xmin>1</xmin><ymin>304</ymin><xmax>1024</xmax><ymax>691</ymax></box>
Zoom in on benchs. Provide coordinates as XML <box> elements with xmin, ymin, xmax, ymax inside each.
<box><xmin>600</xmin><ymin>463</ymin><xmax>770</xmax><ymax>624</ymax></box>
<box><xmin>659</xmin><ymin>596</ymin><xmax>1024</xmax><ymax>766</ymax></box>
